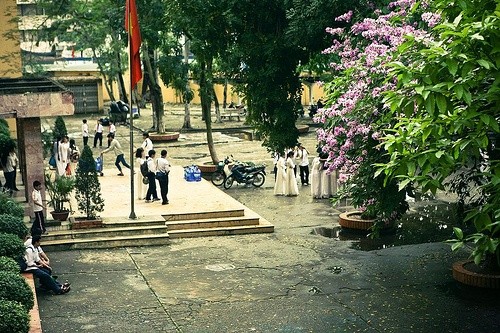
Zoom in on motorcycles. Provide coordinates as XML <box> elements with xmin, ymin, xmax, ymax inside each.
<box><xmin>210</xmin><ymin>154</ymin><xmax>268</xmax><ymax>190</ymax></box>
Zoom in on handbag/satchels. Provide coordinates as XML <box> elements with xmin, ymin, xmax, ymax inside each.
<box><xmin>49</xmin><ymin>156</ymin><xmax>56</xmax><ymax>166</ymax></box>
<box><xmin>156</xmin><ymin>172</ymin><xmax>165</xmax><ymax>179</ymax></box>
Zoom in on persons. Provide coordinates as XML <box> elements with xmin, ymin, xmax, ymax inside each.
<box><xmin>126</xmin><ymin>0</ymin><xmax>142</xmax><ymax>90</ymax></box>
<box><xmin>31</xmin><ymin>180</ymin><xmax>49</xmax><ymax>235</ymax></box>
<box><xmin>297</xmin><ymin>143</ymin><xmax>311</xmax><ymax>186</ymax></box>
<box><xmin>136</xmin><ymin>133</ymin><xmax>171</xmax><ymax>205</ymax></box>
<box><xmin>23</xmin><ymin>228</ymin><xmax>71</xmax><ymax>294</ymax></box>
<box><xmin>52</xmin><ymin>136</ymin><xmax>80</xmax><ymax>181</ymax></box>
<box><xmin>82</xmin><ymin>119</ymin><xmax>89</xmax><ymax>145</ymax></box>
<box><xmin>273</xmin><ymin>144</ymin><xmax>297</xmax><ymax>196</ymax></box>
<box><xmin>311</xmin><ymin>152</ymin><xmax>341</xmax><ymax>199</ymax></box>
<box><xmin>107</xmin><ymin>120</ymin><xmax>115</xmax><ymax>148</ymax></box>
<box><xmin>93</xmin><ymin>120</ymin><xmax>104</xmax><ymax>148</ymax></box>
<box><xmin>1</xmin><ymin>142</ymin><xmax>20</xmax><ymax>193</ymax></box>
<box><xmin>102</xmin><ymin>133</ymin><xmax>136</xmax><ymax>176</ymax></box>
<box><xmin>310</xmin><ymin>105</ymin><xmax>316</xmax><ymax>118</ymax></box>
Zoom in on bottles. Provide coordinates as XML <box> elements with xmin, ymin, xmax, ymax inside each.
<box><xmin>95</xmin><ymin>157</ymin><xmax>102</xmax><ymax>171</ymax></box>
<box><xmin>183</xmin><ymin>164</ymin><xmax>200</xmax><ymax>181</ymax></box>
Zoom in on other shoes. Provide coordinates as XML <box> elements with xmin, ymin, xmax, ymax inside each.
<box><xmin>117</xmin><ymin>173</ymin><xmax>124</xmax><ymax>176</ymax></box>
<box><xmin>43</xmin><ymin>231</ymin><xmax>49</xmax><ymax>235</ymax></box>
<box><xmin>163</xmin><ymin>202</ymin><xmax>169</xmax><ymax>205</ymax></box>
<box><xmin>60</xmin><ymin>284</ymin><xmax>70</xmax><ymax>294</ymax></box>
<box><xmin>145</xmin><ymin>198</ymin><xmax>152</xmax><ymax>200</ymax></box>
<box><xmin>14</xmin><ymin>188</ymin><xmax>20</xmax><ymax>191</ymax></box>
<box><xmin>153</xmin><ymin>198</ymin><xmax>161</xmax><ymax>200</ymax></box>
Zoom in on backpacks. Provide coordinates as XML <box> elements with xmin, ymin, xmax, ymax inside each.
<box><xmin>19</xmin><ymin>247</ymin><xmax>33</xmax><ymax>270</ymax></box>
<box><xmin>70</xmin><ymin>147</ymin><xmax>80</xmax><ymax>163</ymax></box>
<box><xmin>140</xmin><ymin>159</ymin><xmax>151</xmax><ymax>177</ymax></box>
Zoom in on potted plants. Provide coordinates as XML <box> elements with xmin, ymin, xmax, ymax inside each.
<box><xmin>69</xmin><ymin>145</ymin><xmax>105</xmax><ymax>229</ymax></box>
<box><xmin>45</xmin><ymin>174</ymin><xmax>74</xmax><ymax>221</ymax></box>
